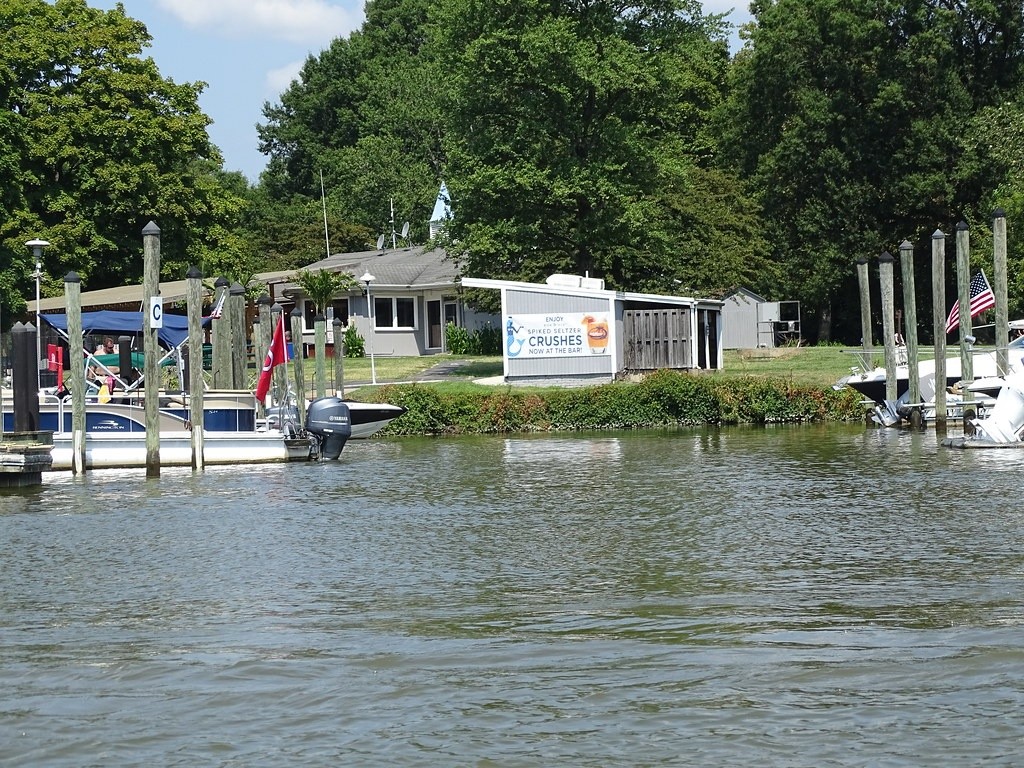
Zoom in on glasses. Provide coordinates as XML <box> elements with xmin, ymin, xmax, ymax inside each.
<box><xmin>107</xmin><ymin>345</ymin><xmax>114</xmax><ymax>349</ymax></box>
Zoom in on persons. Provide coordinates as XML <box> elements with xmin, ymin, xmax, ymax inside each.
<box><xmin>89</xmin><ymin>337</ymin><xmax>120</xmax><ymax>387</ymax></box>
<box><xmin>895</xmin><ymin>329</ymin><xmax>904</xmax><ymax>345</ymax></box>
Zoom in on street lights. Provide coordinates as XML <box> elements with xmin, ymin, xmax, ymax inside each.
<box><xmin>25</xmin><ymin>238</ymin><xmax>50</xmax><ymax>393</ymax></box>
<box><xmin>359</xmin><ymin>266</ymin><xmax>376</xmax><ymax>385</ymax></box>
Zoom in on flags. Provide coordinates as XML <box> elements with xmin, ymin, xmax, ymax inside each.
<box><xmin>944</xmin><ymin>271</ymin><xmax>995</xmax><ymax>335</ymax></box>
<box><xmin>256</xmin><ymin>316</ymin><xmax>290</xmax><ymax>403</ymax></box>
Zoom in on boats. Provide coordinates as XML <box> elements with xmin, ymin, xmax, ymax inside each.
<box><xmin>1</xmin><ymin>388</ymin><xmax>409</xmax><ymax>469</ymax></box>
<box><xmin>846</xmin><ymin>319</ymin><xmax>1024</xmax><ymax>421</ymax></box>
